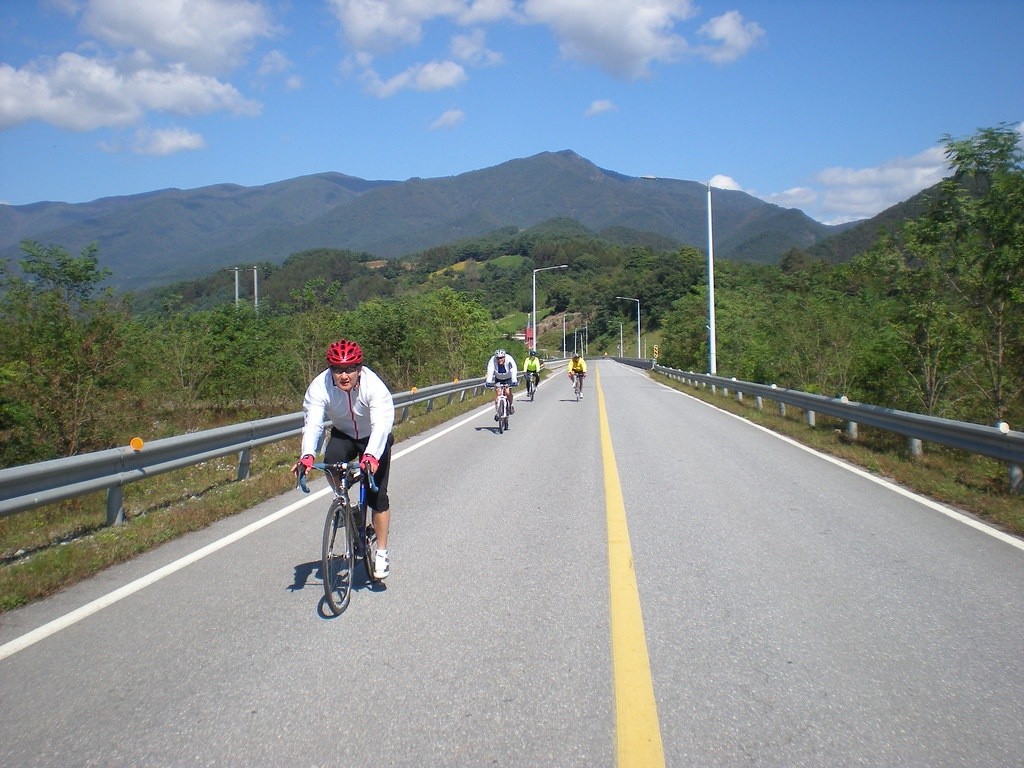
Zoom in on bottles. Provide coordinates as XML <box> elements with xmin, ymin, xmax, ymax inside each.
<box><xmin>350</xmin><ymin>502</ymin><xmax>362</xmax><ymax>528</ymax></box>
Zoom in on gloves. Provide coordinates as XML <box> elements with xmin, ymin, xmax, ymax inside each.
<box><xmin>512</xmin><ymin>382</ymin><xmax>518</xmax><ymax>387</ymax></box>
<box><xmin>583</xmin><ymin>373</ymin><xmax>586</xmax><ymax>377</ymax></box>
<box><xmin>486</xmin><ymin>383</ymin><xmax>491</xmax><ymax>387</ymax></box>
<box><xmin>536</xmin><ymin>372</ymin><xmax>540</xmax><ymax>376</ymax></box>
<box><xmin>568</xmin><ymin>373</ymin><xmax>571</xmax><ymax>377</ymax></box>
<box><xmin>524</xmin><ymin>372</ymin><xmax>527</xmax><ymax>378</ymax></box>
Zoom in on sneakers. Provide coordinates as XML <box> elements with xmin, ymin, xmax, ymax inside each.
<box><xmin>331</xmin><ymin>502</ymin><xmax>351</xmax><ymax>528</ymax></box>
<box><xmin>373</xmin><ymin>551</ymin><xmax>390</xmax><ymax>578</ymax></box>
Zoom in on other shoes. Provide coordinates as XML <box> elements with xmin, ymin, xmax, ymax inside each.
<box><xmin>510</xmin><ymin>406</ymin><xmax>514</xmax><ymax>414</ymax></box>
<box><xmin>495</xmin><ymin>414</ymin><xmax>499</xmax><ymax>422</ymax></box>
<box><xmin>580</xmin><ymin>392</ymin><xmax>583</xmax><ymax>398</ymax></box>
<box><xmin>573</xmin><ymin>382</ymin><xmax>575</xmax><ymax>388</ymax></box>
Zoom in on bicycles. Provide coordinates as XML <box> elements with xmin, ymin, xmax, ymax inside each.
<box><xmin>484</xmin><ymin>380</ymin><xmax>521</xmax><ymax>434</ymax></box>
<box><xmin>523</xmin><ymin>372</ymin><xmax>540</xmax><ymax>402</ymax></box>
<box><xmin>569</xmin><ymin>371</ymin><xmax>586</xmax><ymax>402</ymax></box>
<box><xmin>294</xmin><ymin>459</ymin><xmax>389</xmax><ymax>617</ymax></box>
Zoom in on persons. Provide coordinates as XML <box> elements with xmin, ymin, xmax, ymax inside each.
<box><xmin>523</xmin><ymin>351</ymin><xmax>540</xmax><ymax>397</ymax></box>
<box><xmin>486</xmin><ymin>349</ymin><xmax>517</xmax><ymax>421</ymax></box>
<box><xmin>291</xmin><ymin>338</ymin><xmax>394</xmax><ymax>579</ymax></box>
<box><xmin>569</xmin><ymin>354</ymin><xmax>587</xmax><ymax>398</ymax></box>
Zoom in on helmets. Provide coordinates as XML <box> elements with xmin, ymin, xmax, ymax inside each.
<box><xmin>531</xmin><ymin>351</ymin><xmax>536</xmax><ymax>356</ymax></box>
<box><xmin>573</xmin><ymin>353</ymin><xmax>580</xmax><ymax>359</ymax></box>
<box><xmin>327</xmin><ymin>339</ymin><xmax>363</xmax><ymax>366</ymax></box>
<box><xmin>496</xmin><ymin>348</ymin><xmax>505</xmax><ymax>358</ymax></box>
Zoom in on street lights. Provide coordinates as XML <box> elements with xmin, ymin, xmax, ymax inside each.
<box><xmin>563</xmin><ymin>312</ymin><xmax>581</xmax><ymax>359</ymax></box>
<box><xmin>532</xmin><ymin>264</ymin><xmax>569</xmax><ymax>351</ymax></box>
<box><xmin>527</xmin><ymin>309</ymin><xmax>541</xmax><ymax>340</ymax></box>
<box><xmin>608</xmin><ymin>321</ymin><xmax>624</xmax><ymax>357</ymax></box>
<box><xmin>640</xmin><ymin>174</ymin><xmax>717</xmax><ymax>377</ymax></box>
<box><xmin>615</xmin><ymin>296</ymin><xmax>642</xmax><ymax>360</ymax></box>
<box><xmin>574</xmin><ymin>325</ymin><xmax>582</xmax><ymax>354</ymax></box>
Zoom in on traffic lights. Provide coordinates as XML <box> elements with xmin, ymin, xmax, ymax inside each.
<box><xmin>653</xmin><ymin>345</ymin><xmax>658</xmax><ymax>358</ymax></box>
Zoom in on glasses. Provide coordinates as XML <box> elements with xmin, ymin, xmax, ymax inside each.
<box><xmin>330</xmin><ymin>364</ymin><xmax>360</xmax><ymax>374</ymax></box>
<box><xmin>497</xmin><ymin>356</ymin><xmax>505</xmax><ymax>360</ymax></box>
